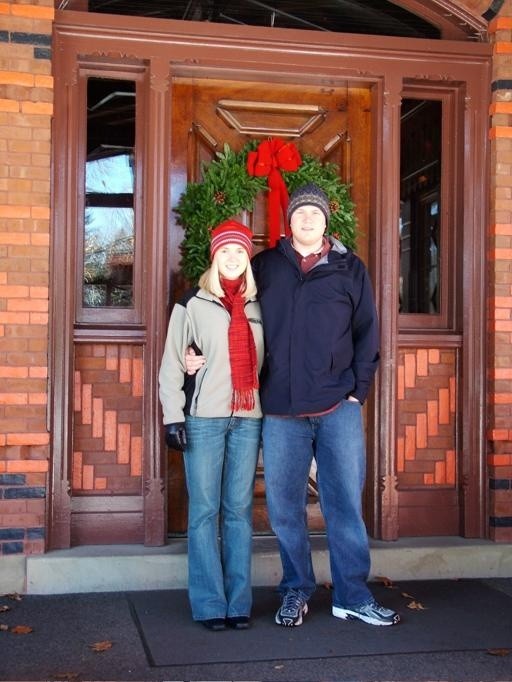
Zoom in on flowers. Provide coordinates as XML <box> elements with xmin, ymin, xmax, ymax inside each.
<box><xmin>172</xmin><ymin>137</ymin><xmax>368</xmax><ymax>294</ymax></box>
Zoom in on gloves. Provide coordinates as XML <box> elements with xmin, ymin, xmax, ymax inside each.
<box><xmin>162</xmin><ymin>421</ymin><xmax>189</xmax><ymax>454</ymax></box>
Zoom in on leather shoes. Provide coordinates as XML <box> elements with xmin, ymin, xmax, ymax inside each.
<box><xmin>202</xmin><ymin>616</ymin><xmax>250</xmax><ymax>631</ymax></box>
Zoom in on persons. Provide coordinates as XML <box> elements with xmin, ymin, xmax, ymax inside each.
<box><xmin>158</xmin><ymin>220</ymin><xmax>265</xmax><ymax>632</ymax></box>
<box><xmin>183</xmin><ymin>184</ymin><xmax>401</xmax><ymax>628</ymax></box>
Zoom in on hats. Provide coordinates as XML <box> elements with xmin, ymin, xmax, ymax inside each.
<box><xmin>210</xmin><ymin>220</ymin><xmax>255</xmax><ymax>268</ymax></box>
<box><xmin>287</xmin><ymin>181</ymin><xmax>331</xmax><ymax>230</ymax></box>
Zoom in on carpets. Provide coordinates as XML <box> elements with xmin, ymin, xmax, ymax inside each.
<box><xmin>124</xmin><ymin>578</ymin><xmax>512</xmax><ymax>667</ymax></box>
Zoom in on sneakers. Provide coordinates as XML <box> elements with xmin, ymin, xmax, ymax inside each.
<box><xmin>330</xmin><ymin>592</ymin><xmax>400</xmax><ymax>629</ymax></box>
<box><xmin>275</xmin><ymin>585</ymin><xmax>314</xmax><ymax>628</ymax></box>
